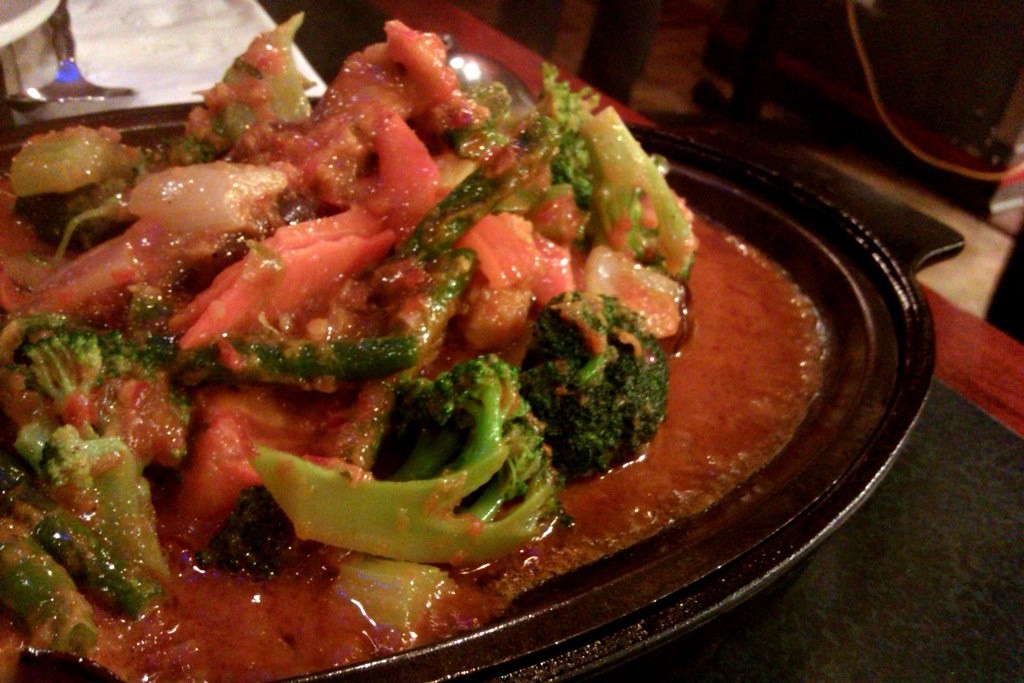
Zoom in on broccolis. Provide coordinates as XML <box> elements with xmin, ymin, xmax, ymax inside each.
<box><xmin>0</xmin><ymin>13</ymin><xmax>698</xmax><ymax>651</ymax></box>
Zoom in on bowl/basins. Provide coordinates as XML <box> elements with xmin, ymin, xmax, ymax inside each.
<box><xmin>0</xmin><ymin>96</ymin><xmax>936</xmax><ymax>683</ymax></box>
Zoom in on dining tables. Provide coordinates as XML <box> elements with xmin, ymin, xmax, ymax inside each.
<box><xmin>0</xmin><ymin>0</ymin><xmax>1024</xmax><ymax>683</ymax></box>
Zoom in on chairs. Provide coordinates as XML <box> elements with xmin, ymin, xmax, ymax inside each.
<box><xmin>636</xmin><ymin>0</ymin><xmax>1024</xmax><ymax>324</ymax></box>
